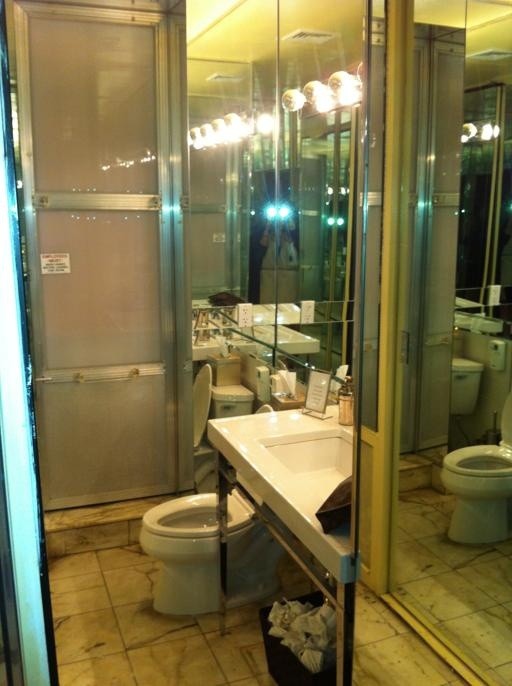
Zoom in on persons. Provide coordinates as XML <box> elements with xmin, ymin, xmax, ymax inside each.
<box><xmin>277</xmin><ymin>201</ymin><xmax>300</xmax><ymax>303</ymax></box>
<box><xmin>253</xmin><ymin>199</ymin><xmax>274</xmax><ymax>303</ymax></box>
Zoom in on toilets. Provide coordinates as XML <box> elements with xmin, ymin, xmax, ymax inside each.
<box><xmin>138</xmin><ymin>405</ymin><xmax>323</xmax><ymax>616</ymax></box>
<box><xmin>440</xmin><ymin>357</ymin><xmax>512</xmax><ymax>544</ymax></box>
<box><xmin>192</xmin><ymin>364</ymin><xmax>255</xmax><ymax>474</ymax></box>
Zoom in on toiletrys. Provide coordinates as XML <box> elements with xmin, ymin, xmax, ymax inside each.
<box><xmin>339</xmin><ymin>376</ymin><xmax>354</xmax><ymax>426</ymax></box>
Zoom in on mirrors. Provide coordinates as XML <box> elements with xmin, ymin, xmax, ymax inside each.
<box><xmin>387</xmin><ymin>0</ymin><xmax>512</xmax><ymax>686</ymax></box>
<box><xmin>185</xmin><ymin>1</ymin><xmax>367</xmax><ymax>493</ymax></box>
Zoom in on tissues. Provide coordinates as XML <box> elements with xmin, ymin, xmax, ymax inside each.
<box><xmin>271</xmin><ymin>370</ymin><xmax>306</xmax><ymax>411</ymax></box>
<box><xmin>207</xmin><ymin>339</ymin><xmax>242</xmax><ymax>387</ymax></box>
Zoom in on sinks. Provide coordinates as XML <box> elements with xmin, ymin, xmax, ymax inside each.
<box><xmin>207</xmin><ymin>406</ymin><xmax>355</xmax><ymax>583</ymax></box>
<box><xmin>192</xmin><ymin>320</ymin><xmax>320</xmax><ymax>362</ymax></box>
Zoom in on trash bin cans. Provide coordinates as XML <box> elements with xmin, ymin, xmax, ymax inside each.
<box><xmin>259</xmin><ymin>590</ymin><xmax>337</xmax><ymax>686</ymax></box>
